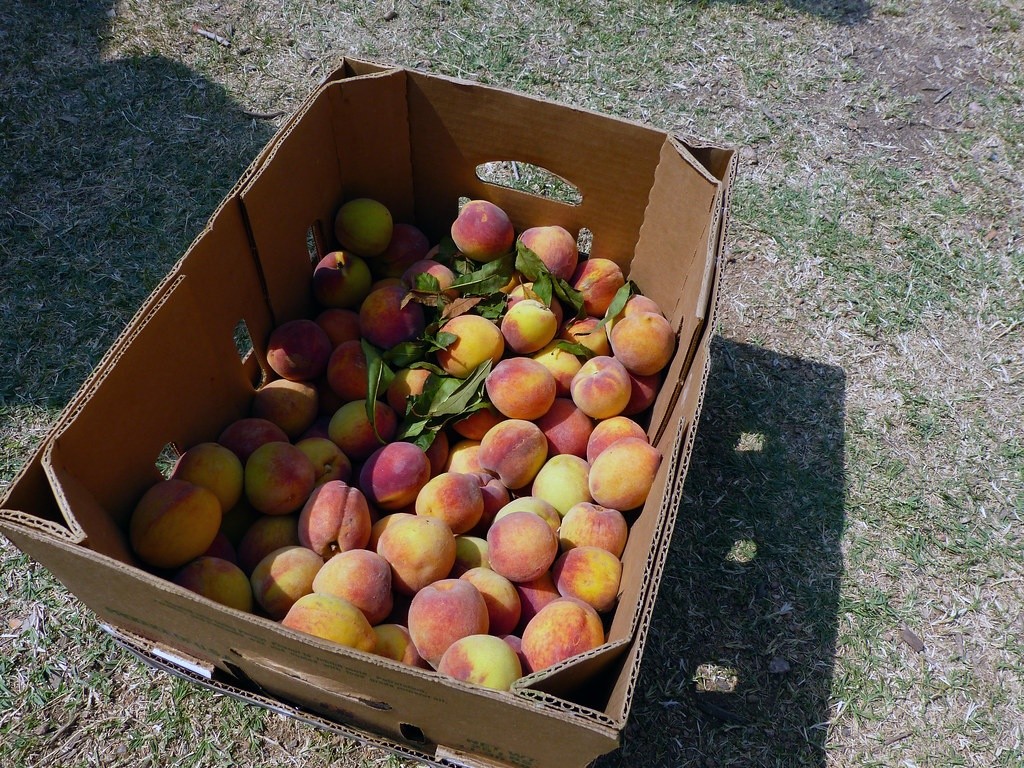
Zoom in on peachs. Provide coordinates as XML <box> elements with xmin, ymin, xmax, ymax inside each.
<box><xmin>123</xmin><ymin>196</ymin><xmax>677</xmax><ymax>690</ymax></box>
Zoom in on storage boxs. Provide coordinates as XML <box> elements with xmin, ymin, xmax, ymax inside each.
<box><xmin>0</xmin><ymin>56</ymin><xmax>741</xmax><ymax>767</ymax></box>
